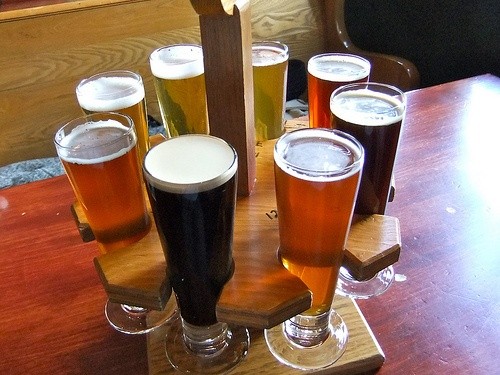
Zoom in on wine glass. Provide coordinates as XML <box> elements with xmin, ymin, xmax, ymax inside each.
<box><xmin>141</xmin><ymin>133</ymin><xmax>250</xmax><ymax>375</ymax></box>
<box><xmin>264</xmin><ymin>128</ymin><xmax>365</xmax><ymax>369</ymax></box>
<box><xmin>53</xmin><ymin>112</ymin><xmax>176</xmax><ymax>334</ymax></box>
<box><xmin>330</xmin><ymin>82</ymin><xmax>406</xmax><ymax>299</ymax></box>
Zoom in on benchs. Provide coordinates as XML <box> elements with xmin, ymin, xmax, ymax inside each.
<box><xmin>0</xmin><ymin>0</ymin><xmax>420</xmax><ymax>188</ymax></box>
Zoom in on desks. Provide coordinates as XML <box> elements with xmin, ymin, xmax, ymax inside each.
<box><xmin>0</xmin><ymin>73</ymin><xmax>500</xmax><ymax>375</ymax></box>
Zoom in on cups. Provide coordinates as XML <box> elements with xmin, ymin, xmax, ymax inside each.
<box><xmin>252</xmin><ymin>41</ymin><xmax>289</xmax><ymax>141</ymax></box>
<box><xmin>75</xmin><ymin>70</ymin><xmax>147</xmax><ymax>152</ymax></box>
<box><xmin>307</xmin><ymin>53</ymin><xmax>370</xmax><ymax>128</ymax></box>
